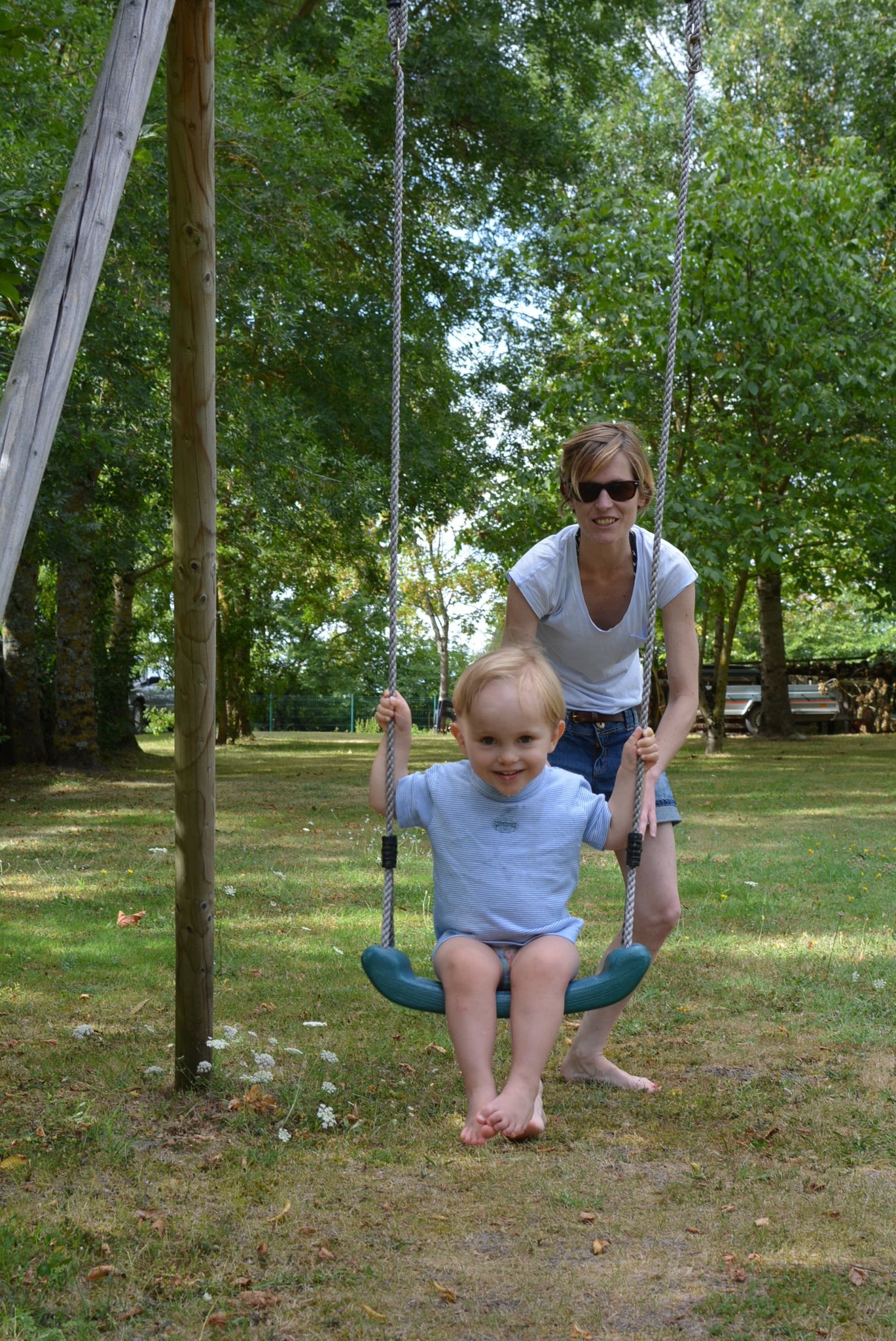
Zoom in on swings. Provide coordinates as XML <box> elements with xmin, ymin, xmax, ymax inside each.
<box><xmin>357</xmin><ymin>1</ymin><xmax>707</xmax><ymax>1020</ymax></box>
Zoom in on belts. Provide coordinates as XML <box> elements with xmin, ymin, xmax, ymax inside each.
<box><xmin>568</xmin><ymin>706</ymin><xmax>641</xmax><ymax>723</ymax></box>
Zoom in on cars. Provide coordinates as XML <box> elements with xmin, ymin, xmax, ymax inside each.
<box><xmin>126</xmin><ymin>675</ymin><xmax>174</xmax><ymax>735</ymax></box>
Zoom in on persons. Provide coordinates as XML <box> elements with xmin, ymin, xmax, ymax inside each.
<box><xmin>366</xmin><ymin>646</ymin><xmax>660</xmax><ymax>1148</ymax></box>
<box><xmin>498</xmin><ymin>423</ymin><xmax>695</xmax><ymax>1095</ymax></box>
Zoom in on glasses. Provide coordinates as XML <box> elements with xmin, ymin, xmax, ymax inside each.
<box><xmin>569</xmin><ymin>480</ymin><xmax>639</xmax><ymax>503</ymax></box>
